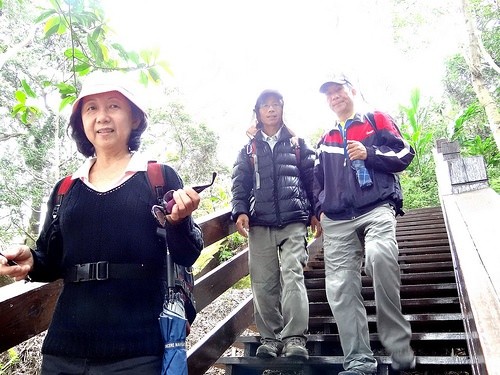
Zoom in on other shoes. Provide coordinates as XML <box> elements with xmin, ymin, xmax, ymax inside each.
<box><xmin>338</xmin><ymin>368</ymin><xmax>373</xmax><ymax>375</ymax></box>
<box><xmin>391</xmin><ymin>350</ymin><xmax>416</xmax><ymax>370</ymax></box>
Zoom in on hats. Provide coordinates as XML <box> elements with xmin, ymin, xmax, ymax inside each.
<box><xmin>70</xmin><ymin>71</ymin><xmax>151</xmax><ymax>118</ymax></box>
<box><xmin>256</xmin><ymin>89</ymin><xmax>283</xmax><ymax>101</ymax></box>
<box><xmin>318</xmin><ymin>75</ymin><xmax>350</xmax><ymax>91</ymax></box>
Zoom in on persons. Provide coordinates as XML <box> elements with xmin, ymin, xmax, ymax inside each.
<box><xmin>312</xmin><ymin>74</ymin><xmax>417</xmax><ymax>375</ymax></box>
<box><xmin>0</xmin><ymin>71</ymin><xmax>203</xmax><ymax>374</ymax></box>
<box><xmin>230</xmin><ymin>89</ymin><xmax>322</xmax><ymax>360</ymax></box>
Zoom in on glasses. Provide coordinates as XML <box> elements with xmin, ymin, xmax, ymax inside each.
<box><xmin>151</xmin><ymin>172</ymin><xmax>217</xmax><ymax>227</ymax></box>
<box><xmin>261</xmin><ymin>103</ymin><xmax>281</xmax><ymax>108</ymax></box>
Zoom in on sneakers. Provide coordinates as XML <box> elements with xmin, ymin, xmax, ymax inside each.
<box><xmin>256</xmin><ymin>342</ymin><xmax>279</xmax><ymax>357</ymax></box>
<box><xmin>280</xmin><ymin>338</ymin><xmax>310</xmax><ymax>359</ymax></box>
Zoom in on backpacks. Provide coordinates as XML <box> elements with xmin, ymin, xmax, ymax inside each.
<box><xmin>50</xmin><ymin>160</ymin><xmax>197</xmax><ymax>326</ymax></box>
